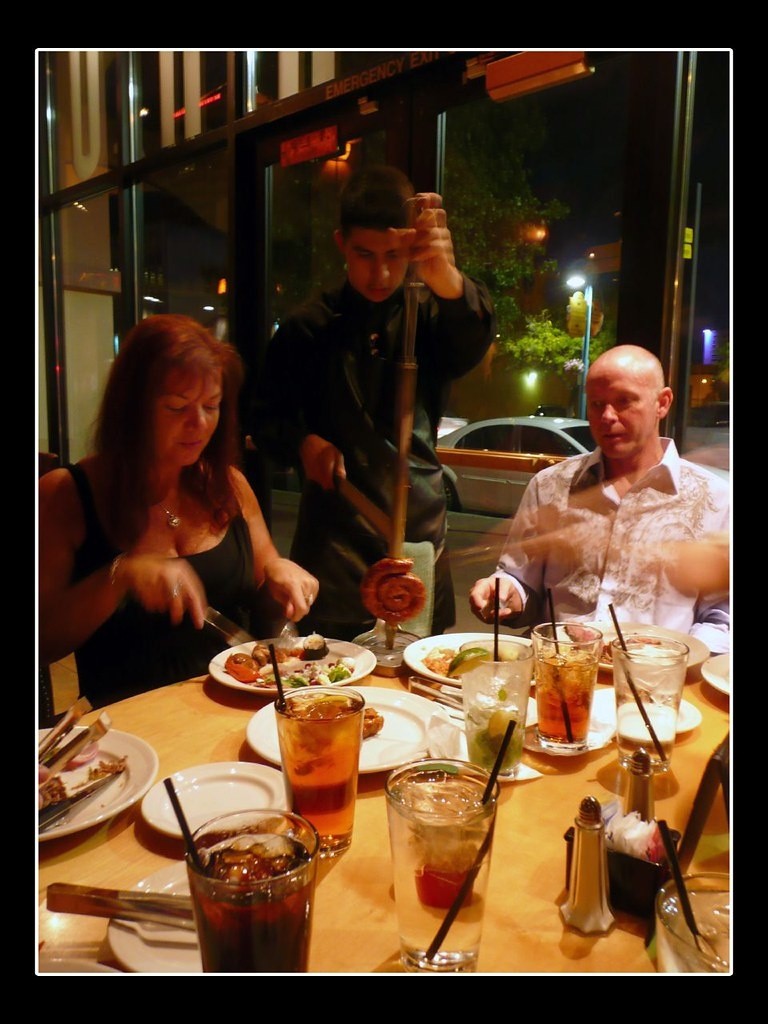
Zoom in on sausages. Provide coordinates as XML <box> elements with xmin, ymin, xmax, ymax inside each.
<box><xmin>359</xmin><ymin>558</ymin><xmax>426</xmax><ymax>624</ymax></box>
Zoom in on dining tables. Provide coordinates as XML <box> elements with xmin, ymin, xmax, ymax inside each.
<box><xmin>38</xmin><ymin>673</ymin><xmax>730</xmax><ymax>975</ymax></box>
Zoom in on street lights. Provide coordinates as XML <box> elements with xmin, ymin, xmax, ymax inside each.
<box><xmin>567</xmin><ymin>276</ymin><xmax>594</xmax><ymax>420</ymax></box>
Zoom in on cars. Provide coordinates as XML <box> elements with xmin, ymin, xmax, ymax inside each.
<box><xmin>436</xmin><ymin>417</ymin><xmax>596</xmax><ymax>516</ymax></box>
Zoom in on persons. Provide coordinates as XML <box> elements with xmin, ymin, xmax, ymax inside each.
<box><xmin>238</xmin><ymin>163</ymin><xmax>499</xmax><ymax>635</ymax></box>
<box><xmin>39</xmin><ymin>314</ymin><xmax>317</xmax><ymax>709</ymax></box>
<box><xmin>470</xmin><ymin>344</ymin><xmax>729</xmax><ymax>636</ymax></box>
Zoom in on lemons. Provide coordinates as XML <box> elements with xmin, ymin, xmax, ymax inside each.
<box><xmin>447</xmin><ymin>648</ymin><xmax>490</xmax><ymax>676</ymax></box>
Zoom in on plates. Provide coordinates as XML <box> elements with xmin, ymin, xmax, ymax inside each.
<box><xmin>208</xmin><ymin>636</ymin><xmax>378</xmax><ymax>696</ymax></box>
<box><xmin>106</xmin><ymin>861</ymin><xmax>203</xmax><ymax>973</ymax></box>
<box><xmin>403</xmin><ymin>622</ymin><xmax>730</xmax><ymax>735</ymax></box>
<box><xmin>141</xmin><ymin>761</ymin><xmax>292</xmax><ymax>842</ymax></box>
<box><xmin>245</xmin><ymin>686</ymin><xmax>448</xmax><ymax>774</ymax></box>
<box><xmin>39</xmin><ymin>722</ymin><xmax>158</xmax><ymax>841</ymax></box>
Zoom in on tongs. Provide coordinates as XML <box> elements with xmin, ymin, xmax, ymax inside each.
<box><xmin>47</xmin><ymin>880</ymin><xmax>196</xmax><ymax>931</ymax></box>
<box><xmin>408</xmin><ymin>676</ymin><xmax>465</xmax><ymax>711</ymax></box>
<box><xmin>39</xmin><ymin>696</ymin><xmax>111</xmax><ymax>779</ymax></box>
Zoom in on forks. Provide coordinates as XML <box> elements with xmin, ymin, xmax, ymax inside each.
<box><xmin>276</xmin><ymin>619</ymin><xmax>300</xmax><ymax>651</ymax></box>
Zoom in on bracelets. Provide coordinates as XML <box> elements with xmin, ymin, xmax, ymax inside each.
<box><xmin>109</xmin><ymin>553</ymin><xmax>125</xmax><ymax>587</ymax></box>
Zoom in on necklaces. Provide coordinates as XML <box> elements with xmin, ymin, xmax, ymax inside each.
<box><xmin>149</xmin><ymin>487</ymin><xmax>181</xmax><ymax>527</ymax></box>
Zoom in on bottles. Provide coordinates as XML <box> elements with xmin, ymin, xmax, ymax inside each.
<box><xmin>560</xmin><ymin>795</ymin><xmax>616</xmax><ymax>935</ymax></box>
<box><xmin>623</xmin><ymin>748</ymin><xmax>659</xmax><ymax>823</ymax></box>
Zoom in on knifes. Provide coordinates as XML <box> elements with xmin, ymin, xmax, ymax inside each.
<box><xmin>39</xmin><ymin>770</ymin><xmax>124</xmax><ymax>830</ymax></box>
<box><xmin>200</xmin><ymin>603</ymin><xmax>262</xmax><ymax>651</ymax></box>
<box><xmin>333</xmin><ymin>471</ymin><xmax>392</xmax><ymax>549</ymax></box>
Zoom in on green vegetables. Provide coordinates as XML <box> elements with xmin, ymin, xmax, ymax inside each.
<box><xmin>265</xmin><ymin>665</ymin><xmax>353</xmax><ymax>688</ymax></box>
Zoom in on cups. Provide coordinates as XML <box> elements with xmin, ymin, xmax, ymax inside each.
<box><xmin>611</xmin><ymin>636</ymin><xmax>690</xmax><ymax>777</ymax></box>
<box><xmin>531</xmin><ymin>622</ymin><xmax>604</xmax><ymax>755</ymax></box>
<box><xmin>387</xmin><ymin>757</ymin><xmax>500</xmax><ymax>971</ymax></box>
<box><xmin>656</xmin><ymin>872</ymin><xmax>730</xmax><ymax>972</ymax></box>
<box><xmin>458</xmin><ymin>640</ymin><xmax>535</xmax><ymax>777</ymax></box>
<box><xmin>185</xmin><ymin>809</ymin><xmax>320</xmax><ymax>972</ymax></box>
<box><xmin>273</xmin><ymin>685</ymin><xmax>365</xmax><ymax>859</ymax></box>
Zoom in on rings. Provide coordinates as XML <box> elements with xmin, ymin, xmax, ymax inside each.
<box><xmin>306</xmin><ymin>594</ymin><xmax>315</xmax><ymax>606</ymax></box>
<box><xmin>170</xmin><ymin>584</ymin><xmax>181</xmax><ymax>599</ymax></box>
